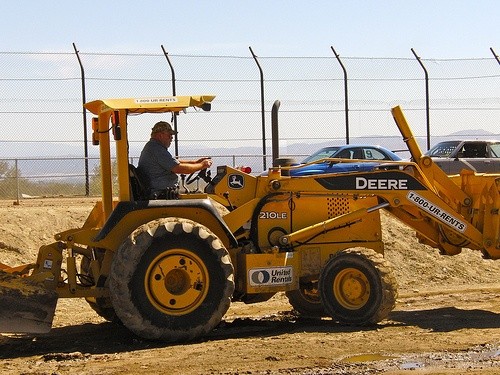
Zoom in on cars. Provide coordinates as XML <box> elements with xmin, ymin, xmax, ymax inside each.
<box><xmin>255</xmin><ymin>143</ymin><xmax>404</xmax><ymax>179</ymax></box>
<box><xmin>410</xmin><ymin>138</ymin><xmax>500</xmax><ymax>175</ymax></box>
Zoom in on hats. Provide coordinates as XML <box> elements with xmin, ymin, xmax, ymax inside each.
<box><xmin>151</xmin><ymin>121</ymin><xmax>178</xmax><ymax>135</ymax></box>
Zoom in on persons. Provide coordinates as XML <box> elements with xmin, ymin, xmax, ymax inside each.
<box><xmin>136</xmin><ymin>122</ymin><xmax>212</xmax><ymax>201</ymax></box>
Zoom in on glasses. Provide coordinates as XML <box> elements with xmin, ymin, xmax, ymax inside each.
<box><xmin>161</xmin><ymin>132</ymin><xmax>172</xmax><ymax>139</ymax></box>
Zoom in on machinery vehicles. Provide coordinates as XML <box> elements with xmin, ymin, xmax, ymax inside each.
<box><xmin>0</xmin><ymin>94</ymin><xmax>500</xmax><ymax>335</ymax></box>
